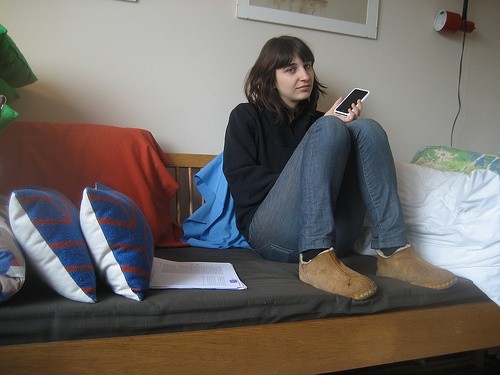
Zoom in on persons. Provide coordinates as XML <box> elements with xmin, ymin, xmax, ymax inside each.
<box><xmin>223</xmin><ymin>35</ymin><xmax>458</xmax><ymax>300</ymax></box>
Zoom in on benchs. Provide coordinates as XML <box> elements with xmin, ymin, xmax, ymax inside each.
<box><xmin>0</xmin><ymin>120</ymin><xmax>500</xmax><ymax>375</ymax></box>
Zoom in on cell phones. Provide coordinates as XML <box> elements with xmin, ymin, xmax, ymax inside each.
<box><xmin>334</xmin><ymin>87</ymin><xmax>370</xmax><ymax>116</ymax></box>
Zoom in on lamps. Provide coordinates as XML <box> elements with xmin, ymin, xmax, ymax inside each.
<box><xmin>433</xmin><ymin>9</ymin><xmax>476</xmax><ymax>35</ymax></box>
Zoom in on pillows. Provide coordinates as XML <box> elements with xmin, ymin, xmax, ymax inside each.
<box><xmin>78</xmin><ymin>182</ymin><xmax>155</xmax><ymax>302</ymax></box>
<box><xmin>355</xmin><ymin>158</ymin><xmax>500</xmax><ymax>307</ymax></box>
<box><xmin>8</xmin><ymin>186</ymin><xmax>98</xmax><ymax>305</ymax></box>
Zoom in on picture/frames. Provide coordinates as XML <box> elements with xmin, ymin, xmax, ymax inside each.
<box><xmin>238</xmin><ymin>0</ymin><xmax>380</xmax><ymax>39</ymax></box>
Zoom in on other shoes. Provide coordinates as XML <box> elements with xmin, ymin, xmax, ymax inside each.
<box><xmin>376</xmin><ymin>244</ymin><xmax>457</xmax><ymax>289</ymax></box>
<box><xmin>298</xmin><ymin>246</ymin><xmax>378</xmax><ymax>300</ymax></box>
